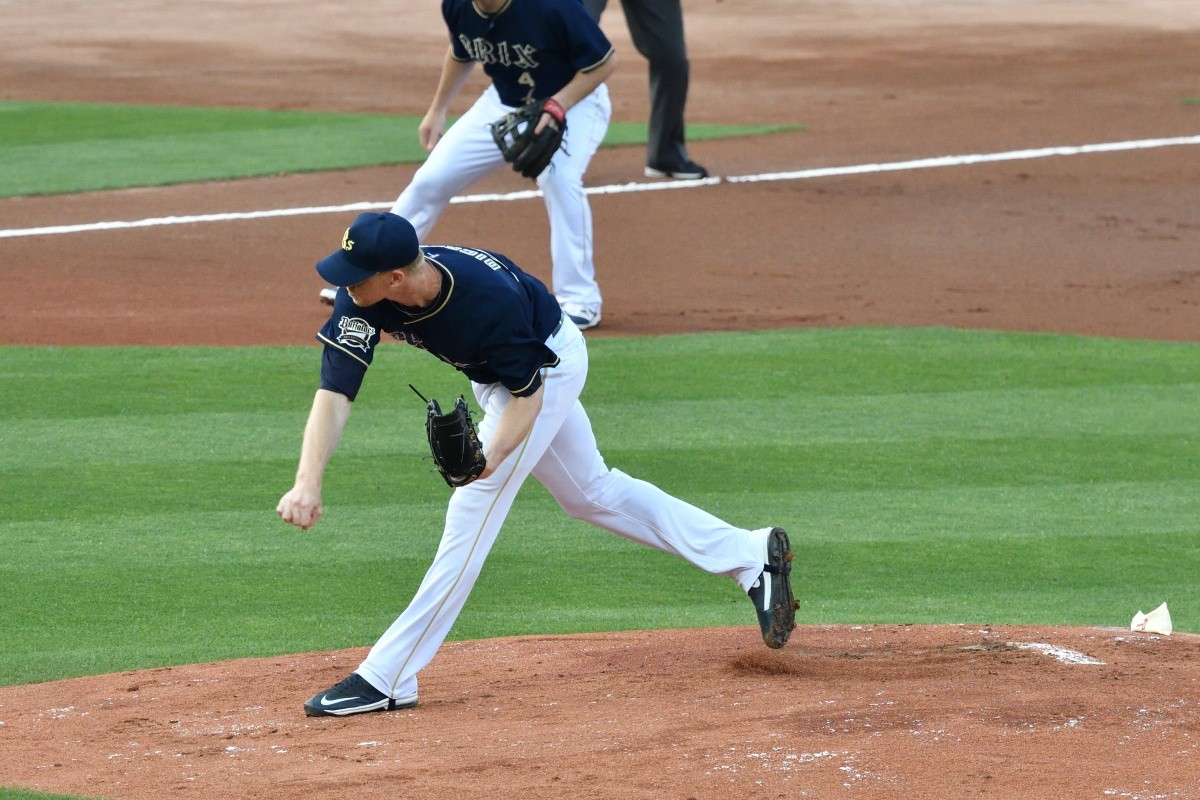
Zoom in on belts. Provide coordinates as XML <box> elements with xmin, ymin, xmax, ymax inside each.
<box><xmin>552</xmin><ymin>311</ymin><xmax>564</xmax><ymax>338</ymax></box>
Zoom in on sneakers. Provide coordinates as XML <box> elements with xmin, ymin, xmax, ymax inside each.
<box><xmin>748</xmin><ymin>528</ymin><xmax>800</xmax><ymax>649</ymax></box>
<box><xmin>320</xmin><ymin>287</ymin><xmax>338</xmax><ymax>304</ymax></box>
<box><xmin>304</xmin><ymin>673</ymin><xmax>419</xmax><ymax>716</ymax></box>
<box><xmin>644</xmin><ymin>161</ymin><xmax>703</xmax><ymax>179</ymax></box>
<box><xmin>561</xmin><ymin>302</ymin><xmax>601</xmax><ymax>329</ymax></box>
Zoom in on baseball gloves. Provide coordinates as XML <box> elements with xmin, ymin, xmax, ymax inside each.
<box><xmin>488</xmin><ymin>98</ymin><xmax>566</xmax><ymax>179</ymax></box>
<box><xmin>423</xmin><ymin>398</ymin><xmax>486</xmax><ymax>489</ymax></box>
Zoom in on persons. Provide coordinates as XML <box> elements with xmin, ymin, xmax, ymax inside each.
<box><xmin>576</xmin><ymin>0</ymin><xmax>708</xmax><ymax>180</ymax></box>
<box><xmin>276</xmin><ymin>210</ymin><xmax>802</xmax><ymax>717</ymax></box>
<box><xmin>320</xmin><ymin>0</ymin><xmax>620</xmax><ymax>332</ymax></box>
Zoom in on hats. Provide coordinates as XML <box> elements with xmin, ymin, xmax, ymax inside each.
<box><xmin>315</xmin><ymin>212</ymin><xmax>419</xmax><ymax>287</ymax></box>
